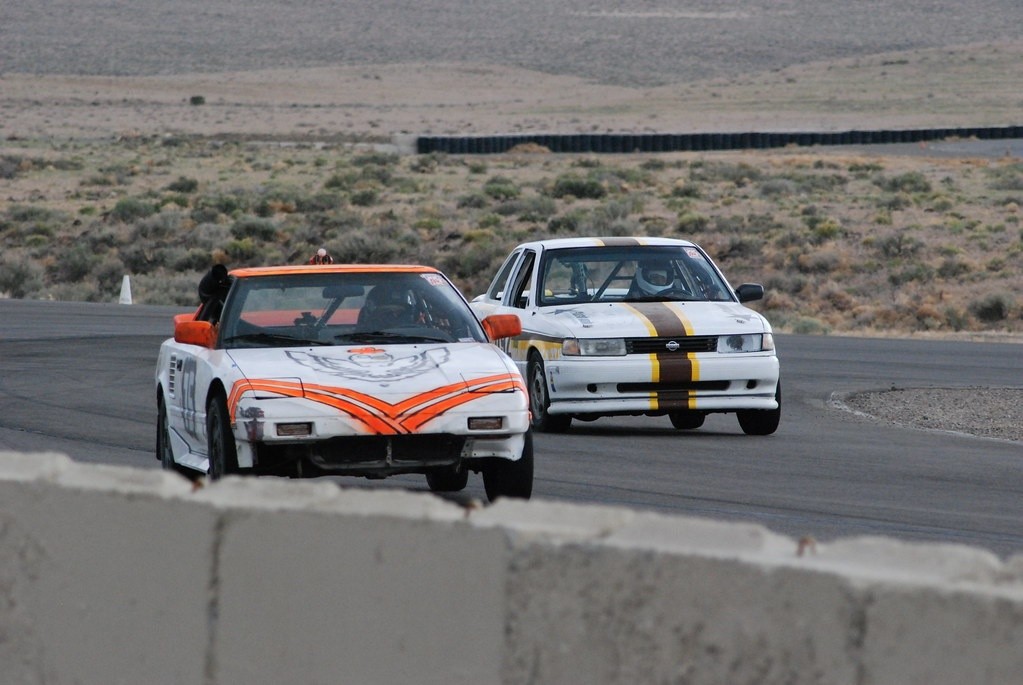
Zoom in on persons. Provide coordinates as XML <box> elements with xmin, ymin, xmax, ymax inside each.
<box><xmin>632</xmin><ymin>260</ymin><xmax>675</xmax><ymax>299</ymax></box>
<box><xmin>364</xmin><ymin>284</ymin><xmax>420</xmax><ymax>333</ymax></box>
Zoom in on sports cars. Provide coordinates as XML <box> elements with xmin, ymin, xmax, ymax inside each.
<box><xmin>155</xmin><ymin>245</ymin><xmax>534</xmax><ymax>506</ymax></box>
<box><xmin>466</xmin><ymin>237</ymin><xmax>780</xmax><ymax>438</ymax></box>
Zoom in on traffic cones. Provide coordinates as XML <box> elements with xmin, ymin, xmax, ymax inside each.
<box><xmin>118</xmin><ymin>275</ymin><xmax>134</xmax><ymax>305</ymax></box>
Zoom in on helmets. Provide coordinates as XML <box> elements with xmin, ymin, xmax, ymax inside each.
<box><xmin>364</xmin><ymin>286</ymin><xmax>416</xmax><ymax>329</ymax></box>
<box><xmin>636</xmin><ymin>258</ymin><xmax>675</xmax><ymax>295</ymax></box>
